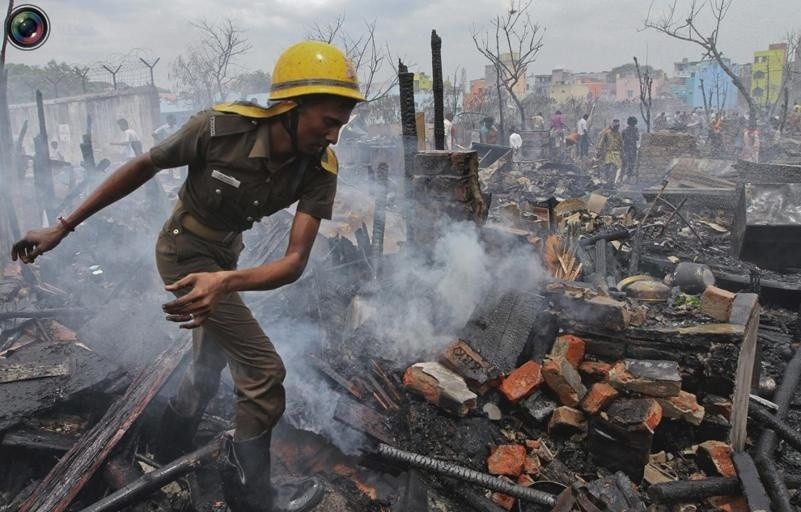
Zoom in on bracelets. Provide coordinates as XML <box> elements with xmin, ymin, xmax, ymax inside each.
<box><xmin>57</xmin><ymin>216</ymin><xmax>74</xmax><ymax>232</ymax></box>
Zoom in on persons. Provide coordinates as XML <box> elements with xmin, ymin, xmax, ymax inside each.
<box><xmin>530</xmin><ymin>110</ymin><xmax>593</xmax><ymax>157</ymax></box>
<box><xmin>149</xmin><ymin>111</ymin><xmax>181</xmax><ymax>177</ymax></box>
<box><xmin>653</xmin><ymin>104</ymin><xmax>800</xmax><ymax>163</ymax></box>
<box><xmin>12</xmin><ymin>38</ymin><xmax>370</xmax><ymax>511</ymax></box>
<box><xmin>507</xmin><ymin>125</ymin><xmax>523</xmax><ymax>161</ymax></box>
<box><xmin>597</xmin><ymin>116</ymin><xmax>640</xmax><ymax>190</ymax></box>
<box><xmin>107</xmin><ymin>118</ymin><xmax>141</xmax><ymax>166</ymax></box>
<box><xmin>443</xmin><ymin>112</ymin><xmax>455</xmax><ymax>151</ymax></box>
<box><xmin>480</xmin><ymin>117</ymin><xmax>498</xmax><ymax>145</ymax></box>
<box><xmin>49</xmin><ymin>138</ymin><xmax>65</xmax><ymax>176</ymax></box>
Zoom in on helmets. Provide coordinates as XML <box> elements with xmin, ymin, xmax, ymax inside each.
<box><xmin>268</xmin><ymin>40</ymin><xmax>366</xmax><ymax>102</ymax></box>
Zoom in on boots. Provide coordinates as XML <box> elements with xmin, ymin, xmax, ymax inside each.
<box><xmin>160</xmin><ymin>394</ymin><xmax>203</xmax><ymax>465</ymax></box>
<box><xmin>223</xmin><ymin>429</ymin><xmax>325</xmax><ymax>512</ymax></box>
